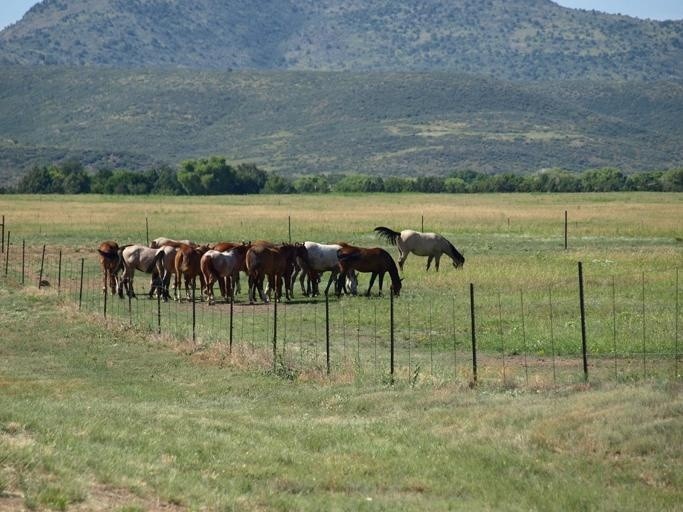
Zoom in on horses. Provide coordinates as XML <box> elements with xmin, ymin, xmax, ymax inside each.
<box><xmin>336</xmin><ymin>245</ymin><xmax>405</xmax><ymax>299</ymax></box>
<box><xmin>97</xmin><ymin>237</ymin><xmax>359</xmax><ymax>306</ymax></box>
<box><xmin>373</xmin><ymin>227</ymin><xmax>465</xmax><ymax>273</ymax></box>
